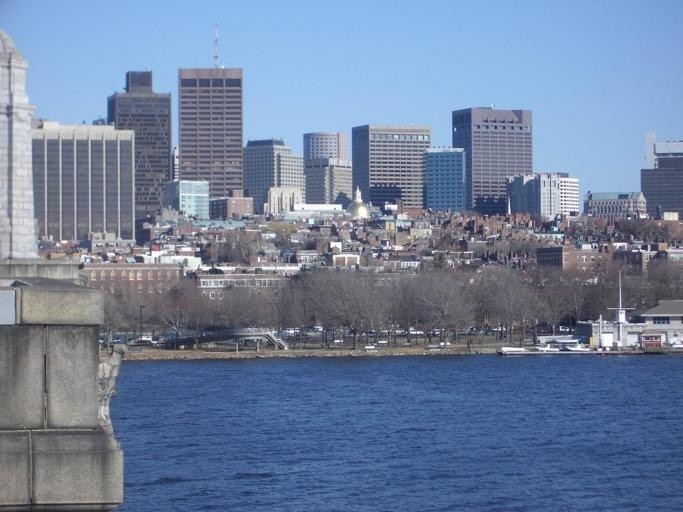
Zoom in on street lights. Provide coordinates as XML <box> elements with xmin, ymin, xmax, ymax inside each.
<box><xmin>139</xmin><ymin>304</ymin><xmax>148</xmax><ymax>344</ymax></box>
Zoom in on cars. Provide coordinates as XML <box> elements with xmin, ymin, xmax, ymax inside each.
<box><xmin>312</xmin><ymin>325</ymin><xmax>324</xmax><ymax>332</ymax></box>
<box><xmin>366</xmin><ymin>326</ymin><xmax>446</xmax><ymax>337</ymax></box>
<box><xmin>282</xmin><ymin>327</ymin><xmax>299</xmax><ymax>336</ymax></box>
<box><xmin>469</xmin><ymin>326</ymin><xmax>528</xmax><ymax>332</ymax></box>
<box><xmin>560</xmin><ymin>325</ymin><xmax>575</xmax><ymax>332</ymax></box>
<box><xmin>98</xmin><ymin>331</ymin><xmax>178</xmax><ymax>345</ymax></box>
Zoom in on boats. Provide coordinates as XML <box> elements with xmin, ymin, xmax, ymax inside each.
<box><xmin>535</xmin><ymin>344</ymin><xmax>560</xmax><ymax>352</ymax></box>
<box><xmin>564</xmin><ymin>342</ymin><xmax>591</xmax><ymax>351</ymax></box>
